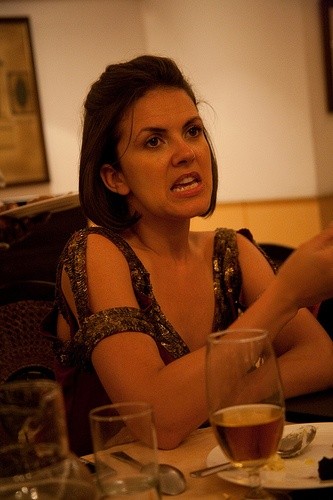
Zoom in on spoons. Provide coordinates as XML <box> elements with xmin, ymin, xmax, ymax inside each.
<box><xmin>111</xmin><ymin>450</ymin><xmax>187</xmax><ymax>495</ymax></box>
<box><xmin>189</xmin><ymin>426</ymin><xmax>317</xmax><ymax>479</ymax></box>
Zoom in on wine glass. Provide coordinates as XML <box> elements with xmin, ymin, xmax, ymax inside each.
<box><xmin>205</xmin><ymin>329</ymin><xmax>285</xmax><ymax>500</ymax></box>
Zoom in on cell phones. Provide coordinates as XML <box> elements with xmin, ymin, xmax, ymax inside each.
<box><xmin>80</xmin><ymin>458</ymin><xmax>116</xmax><ymax>483</ymax></box>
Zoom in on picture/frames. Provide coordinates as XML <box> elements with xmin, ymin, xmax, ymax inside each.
<box><xmin>0</xmin><ymin>17</ymin><xmax>51</xmax><ymax>187</ymax></box>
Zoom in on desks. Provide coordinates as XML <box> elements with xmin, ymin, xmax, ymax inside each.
<box><xmin>0</xmin><ymin>417</ymin><xmax>332</xmax><ymax>500</ymax></box>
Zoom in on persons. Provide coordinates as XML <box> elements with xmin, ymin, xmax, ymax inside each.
<box><xmin>38</xmin><ymin>55</ymin><xmax>333</xmax><ymax>459</ymax></box>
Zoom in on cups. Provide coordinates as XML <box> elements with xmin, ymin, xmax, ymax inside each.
<box><xmin>90</xmin><ymin>403</ymin><xmax>159</xmax><ymax>500</ymax></box>
<box><xmin>0</xmin><ymin>380</ymin><xmax>70</xmax><ymax>500</ymax></box>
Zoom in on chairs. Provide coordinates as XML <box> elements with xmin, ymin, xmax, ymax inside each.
<box><xmin>259</xmin><ymin>241</ymin><xmax>333</xmax><ymax>418</ymax></box>
<box><xmin>0</xmin><ymin>278</ymin><xmax>118</xmax><ymax>458</ymax></box>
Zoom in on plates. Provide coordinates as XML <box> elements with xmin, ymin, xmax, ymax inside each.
<box><xmin>0</xmin><ymin>192</ymin><xmax>82</xmax><ymax>218</ymax></box>
<box><xmin>206</xmin><ymin>422</ymin><xmax>333</xmax><ymax>500</ymax></box>
